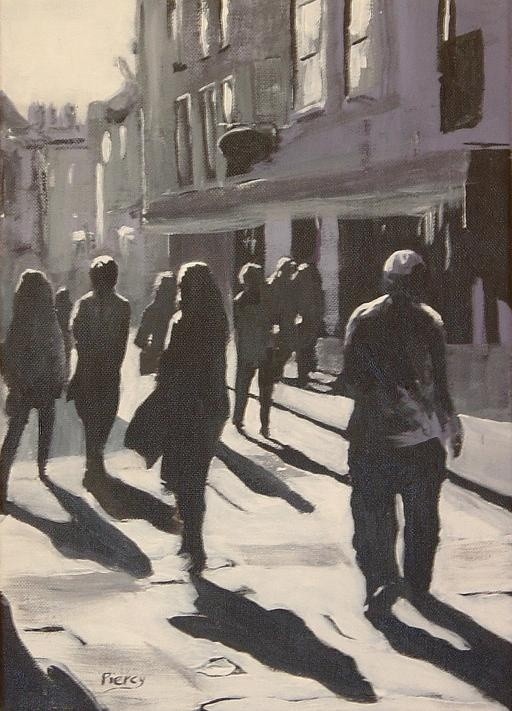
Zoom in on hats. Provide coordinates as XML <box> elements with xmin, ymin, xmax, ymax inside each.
<box><xmin>384</xmin><ymin>249</ymin><xmax>426</xmax><ymax>276</ymax></box>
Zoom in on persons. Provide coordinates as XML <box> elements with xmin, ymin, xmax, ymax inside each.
<box><xmin>3</xmin><ymin>269</ymin><xmax>67</xmax><ymax>503</ymax></box>
<box><xmin>232</xmin><ymin>254</ymin><xmax>323</xmax><ymax>440</ymax></box>
<box><xmin>123</xmin><ymin>261</ymin><xmax>229</xmax><ymax>578</ymax></box>
<box><xmin>134</xmin><ymin>270</ymin><xmax>179</xmax><ymax>381</ymax></box>
<box><xmin>52</xmin><ymin>289</ymin><xmax>73</xmax><ymax>399</ymax></box>
<box><xmin>69</xmin><ymin>254</ymin><xmax>132</xmax><ymax>487</ymax></box>
<box><xmin>332</xmin><ymin>246</ymin><xmax>463</xmax><ymax>620</ymax></box>
